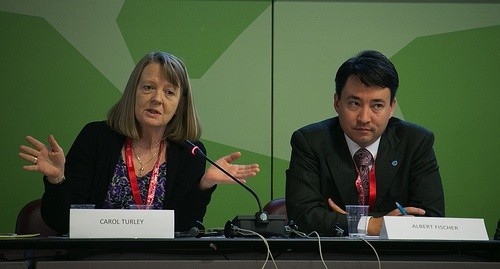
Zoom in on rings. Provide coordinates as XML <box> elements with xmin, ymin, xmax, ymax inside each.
<box><xmin>33</xmin><ymin>158</ymin><xmax>38</xmax><ymax>163</ymax></box>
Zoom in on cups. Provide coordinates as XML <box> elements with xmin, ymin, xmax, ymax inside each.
<box><xmin>130</xmin><ymin>204</ymin><xmax>153</xmax><ymax>210</ymax></box>
<box><xmin>345</xmin><ymin>205</ymin><xmax>369</xmax><ymax>240</ymax></box>
<box><xmin>70</xmin><ymin>205</ymin><xmax>96</xmax><ymax>209</ymax></box>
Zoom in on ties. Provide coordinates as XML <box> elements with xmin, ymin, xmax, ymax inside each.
<box><xmin>354</xmin><ymin>148</ymin><xmax>373</xmax><ymax>205</ymax></box>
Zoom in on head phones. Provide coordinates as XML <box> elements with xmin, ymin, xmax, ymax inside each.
<box><xmin>189</xmin><ymin>220</ymin><xmax>238</xmax><ymax>238</ymax></box>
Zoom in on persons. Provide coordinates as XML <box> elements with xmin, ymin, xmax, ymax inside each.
<box><xmin>285</xmin><ymin>50</ymin><xmax>446</xmax><ymax>235</ymax></box>
<box><xmin>19</xmin><ymin>52</ymin><xmax>261</xmax><ymax>234</ymax></box>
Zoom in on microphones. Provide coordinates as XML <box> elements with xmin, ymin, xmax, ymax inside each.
<box><xmin>184</xmin><ymin>139</ymin><xmax>287</xmax><ymax>237</ymax></box>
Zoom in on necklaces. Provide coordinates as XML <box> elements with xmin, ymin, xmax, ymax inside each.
<box><xmin>134</xmin><ymin>138</ymin><xmax>159</xmax><ymax>176</ymax></box>
